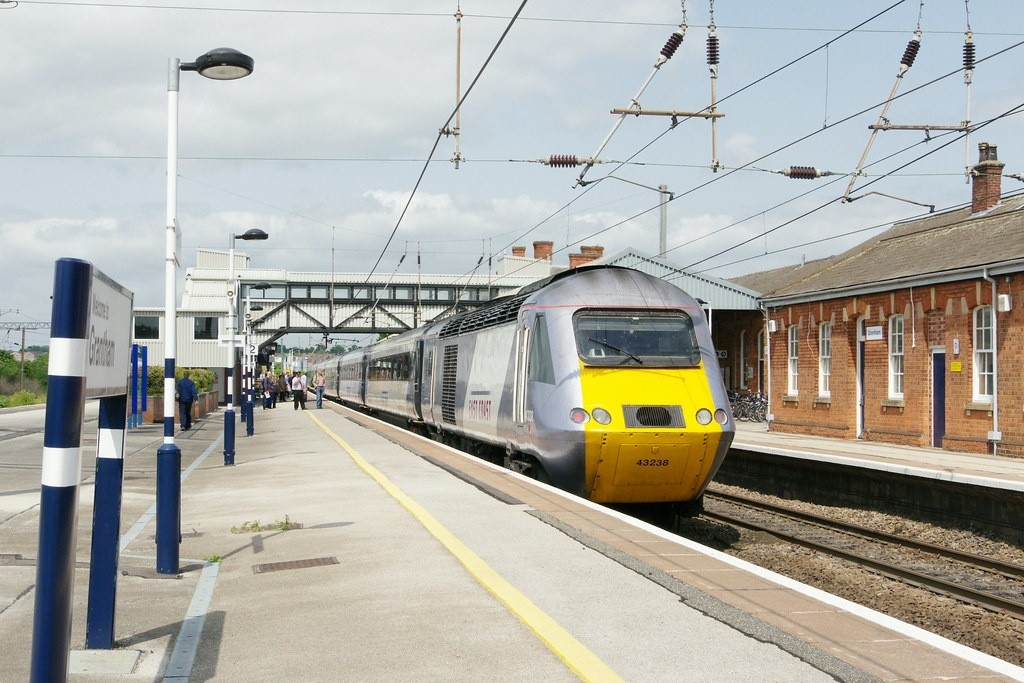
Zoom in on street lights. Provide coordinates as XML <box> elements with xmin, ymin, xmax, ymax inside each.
<box><xmin>156</xmin><ymin>47</ymin><xmax>254</xmax><ymax>574</ymax></box>
<box><xmin>241</xmin><ymin>318</ymin><xmax>265</xmax><ymax>415</ymax></box>
<box><xmin>240</xmin><ymin>306</ymin><xmax>263</xmax><ymax>422</ymax></box>
<box><xmin>223</xmin><ymin>229</ymin><xmax>268</xmax><ymax>465</ymax></box>
<box><xmin>241</xmin><ymin>282</ymin><xmax>272</xmax><ymax>436</ymax></box>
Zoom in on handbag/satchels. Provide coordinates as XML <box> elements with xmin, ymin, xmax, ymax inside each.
<box><xmin>175</xmin><ymin>380</ymin><xmax>181</xmax><ymax>402</ymax></box>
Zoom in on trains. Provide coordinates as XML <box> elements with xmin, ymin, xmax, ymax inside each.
<box><xmin>305</xmin><ymin>265</ymin><xmax>736</xmax><ymax>518</ymax></box>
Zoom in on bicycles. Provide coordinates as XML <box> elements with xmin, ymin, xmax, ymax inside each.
<box><xmin>727</xmin><ymin>389</ymin><xmax>768</xmax><ymax>422</ymax></box>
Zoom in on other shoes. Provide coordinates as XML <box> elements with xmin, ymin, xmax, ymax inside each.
<box><xmin>186</xmin><ymin>426</ymin><xmax>190</xmax><ymax>431</ymax></box>
<box><xmin>181</xmin><ymin>426</ymin><xmax>185</xmax><ymax>431</ymax></box>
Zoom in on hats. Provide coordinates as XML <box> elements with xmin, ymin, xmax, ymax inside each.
<box><xmin>294</xmin><ymin>372</ymin><xmax>301</xmax><ymax>375</ymax></box>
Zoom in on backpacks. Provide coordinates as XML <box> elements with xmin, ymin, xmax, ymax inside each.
<box><xmin>310</xmin><ymin>374</ymin><xmax>324</xmax><ymax>387</ymax></box>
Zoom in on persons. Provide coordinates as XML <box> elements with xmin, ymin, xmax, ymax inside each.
<box><xmin>254</xmin><ymin>381</ymin><xmax>260</xmax><ymax>398</ymax></box>
<box><xmin>311</xmin><ymin>368</ymin><xmax>326</xmax><ymax>409</ymax></box>
<box><xmin>278</xmin><ymin>375</ymin><xmax>288</xmax><ymax>402</ymax></box>
<box><xmin>262</xmin><ymin>372</ymin><xmax>278</xmax><ymax>410</ymax></box>
<box><xmin>290</xmin><ymin>371</ymin><xmax>310</xmax><ymax>410</ymax></box>
<box><xmin>284</xmin><ymin>371</ymin><xmax>290</xmax><ymax>399</ymax></box>
<box><xmin>175</xmin><ymin>371</ymin><xmax>198</xmax><ymax>431</ymax></box>
